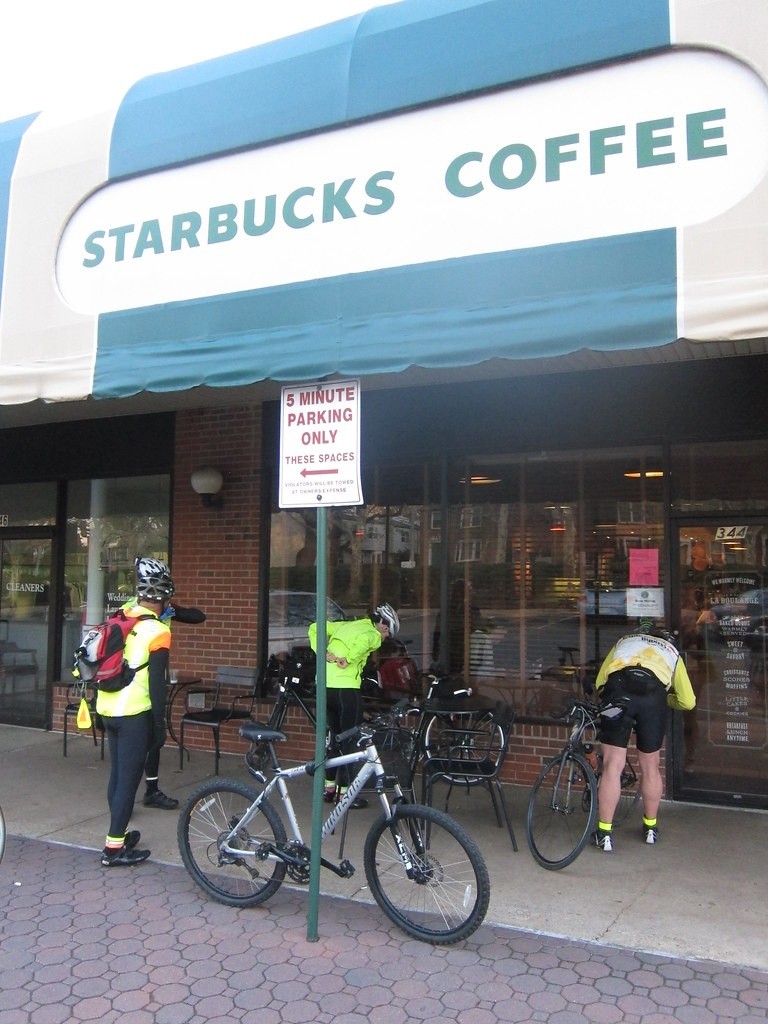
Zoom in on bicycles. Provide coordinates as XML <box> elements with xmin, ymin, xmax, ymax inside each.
<box><xmin>257</xmin><ymin>660</ymin><xmax>418</xmax><ymax>788</ymax></box>
<box><xmin>362</xmin><ymin>670</ymin><xmax>508</xmax><ymax>787</ymax></box>
<box><xmin>391</xmin><ymin>637</ymin><xmax>435</xmax><ymax>661</ymax></box>
<box><xmin>176</xmin><ymin>698</ymin><xmax>490</xmax><ymax>946</ymax></box>
<box><xmin>541</xmin><ymin>645</ymin><xmax>608</xmax><ymax>719</ymax></box>
<box><xmin>525</xmin><ymin>695</ymin><xmax>642</xmax><ymax>871</ymax></box>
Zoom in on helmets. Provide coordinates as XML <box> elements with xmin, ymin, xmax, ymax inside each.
<box><xmin>376</xmin><ymin>602</ymin><xmax>400</xmax><ymax>640</ymax></box>
<box><xmin>134</xmin><ymin>557</ymin><xmax>170</xmax><ymax>578</ymax></box>
<box><xmin>136</xmin><ymin>572</ymin><xmax>175</xmax><ymax>602</ymax></box>
<box><xmin>646</xmin><ymin>626</ymin><xmax>678</xmax><ymax>648</ymax></box>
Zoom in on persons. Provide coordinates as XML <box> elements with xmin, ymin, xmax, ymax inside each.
<box><xmin>589</xmin><ymin>629</ymin><xmax>697</xmax><ymax>851</ymax></box>
<box><xmin>95</xmin><ymin>557</ymin><xmax>206</xmax><ymax>867</ymax></box>
<box><xmin>308</xmin><ymin>603</ymin><xmax>399</xmax><ymax>809</ymax></box>
<box><xmin>431</xmin><ymin>578</ymin><xmax>484</xmax><ymax>676</ymax></box>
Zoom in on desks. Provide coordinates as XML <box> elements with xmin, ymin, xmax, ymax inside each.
<box><xmin>166</xmin><ymin>676</ymin><xmax>202</xmax><ymax>762</ymax></box>
<box><xmin>409</xmin><ymin>697</ymin><xmax>499</xmax><ymax>804</ymax></box>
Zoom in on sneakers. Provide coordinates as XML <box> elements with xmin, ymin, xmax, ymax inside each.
<box><xmin>323</xmin><ymin>789</ymin><xmax>369</xmax><ymax>809</ymax></box>
<box><xmin>642</xmin><ymin>823</ymin><xmax>657</xmax><ymax>844</ymax></box>
<box><xmin>100</xmin><ymin>830</ymin><xmax>149</xmax><ymax>867</ymax></box>
<box><xmin>589</xmin><ymin>829</ymin><xmax>614</xmax><ymax>853</ymax></box>
<box><xmin>143</xmin><ymin>790</ymin><xmax>179</xmax><ymax>810</ymax></box>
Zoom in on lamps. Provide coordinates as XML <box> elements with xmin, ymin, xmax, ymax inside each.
<box><xmin>191</xmin><ymin>466</ymin><xmax>224</xmax><ymax>511</ymax></box>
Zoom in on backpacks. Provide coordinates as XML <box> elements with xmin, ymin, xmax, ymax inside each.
<box><xmin>73</xmin><ymin>608</ymin><xmax>163</xmax><ymax>693</ymax></box>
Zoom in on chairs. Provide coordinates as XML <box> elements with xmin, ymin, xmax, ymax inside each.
<box><xmin>333</xmin><ymin>691</ymin><xmax>430</xmax><ymax>857</ymax></box>
<box><xmin>180</xmin><ymin>665</ymin><xmax>262</xmax><ymax>775</ymax></box>
<box><xmin>420</xmin><ymin>697</ymin><xmax>520</xmax><ymax>852</ymax></box>
<box><xmin>62</xmin><ymin>681</ymin><xmax>108</xmax><ymax>759</ymax></box>
<box><xmin>0</xmin><ymin>619</ymin><xmax>41</xmax><ymax>701</ymax></box>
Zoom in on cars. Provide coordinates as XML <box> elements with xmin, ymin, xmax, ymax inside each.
<box><xmin>268</xmin><ymin>589</ymin><xmax>348</xmax><ymax>667</ymax></box>
<box><xmin>698</xmin><ymin>587</ymin><xmax>768</xmax><ymax>652</ymax></box>
<box><xmin>578</xmin><ymin>586</ymin><xmax>630</xmax><ymax>620</ymax></box>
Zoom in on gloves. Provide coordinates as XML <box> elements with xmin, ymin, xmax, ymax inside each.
<box><xmin>150</xmin><ymin>721</ymin><xmax>167</xmax><ymax>754</ymax></box>
<box><xmin>95</xmin><ymin>712</ymin><xmax>106</xmax><ymax>732</ymax></box>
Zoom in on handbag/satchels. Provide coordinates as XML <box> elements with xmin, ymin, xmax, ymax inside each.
<box><xmin>622</xmin><ymin>665</ymin><xmax>659</xmax><ymax>696</ymax></box>
<box><xmin>76</xmin><ymin>682</ymin><xmax>92</xmax><ymax>728</ymax></box>
<box><xmin>338</xmin><ymin>716</ymin><xmax>419</xmax><ymax>788</ymax></box>
<box><xmin>426</xmin><ymin>677</ymin><xmax>516</xmax><ymax>734</ymax></box>
